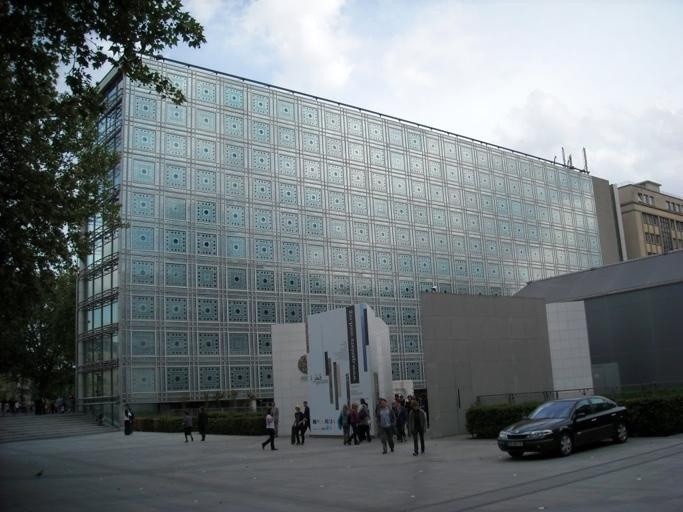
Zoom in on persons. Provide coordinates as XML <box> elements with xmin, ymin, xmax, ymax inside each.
<box><xmin>122</xmin><ymin>403</ymin><xmax>135</xmax><ymax>436</ymax></box>
<box><xmin>337</xmin><ymin>392</ymin><xmax>428</xmax><ymax>456</ymax></box>
<box><xmin>179</xmin><ymin>410</ymin><xmax>194</xmax><ymax>444</ymax></box>
<box><xmin>269</xmin><ymin>402</ymin><xmax>280</xmax><ymax>438</ymax></box>
<box><xmin>261</xmin><ymin>408</ymin><xmax>279</xmax><ymax>451</ymax></box>
<box><xmin>1</xmin><ymin>389</ymin><xmax>76</xmax><ymax>416</ymax></box>
<box><xmin>289</xmin><ymin>405</ymin><xmax>305</xmax><ymax>446</ymax></box>
<box><xmin>299</xmin><ymin>399</ymin><xmax>312</xmax><ymax>445</ymax></box>
<box><xmin>196</xmin><ymin>406</ymin><xmax>209</xmax><ymax>443</ymax></box>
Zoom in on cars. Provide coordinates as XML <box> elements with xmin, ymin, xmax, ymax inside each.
<box><xmin>497</xmin><ymin>395</ymin><xmax>630</xmax><ymax>457</ymax></box>
